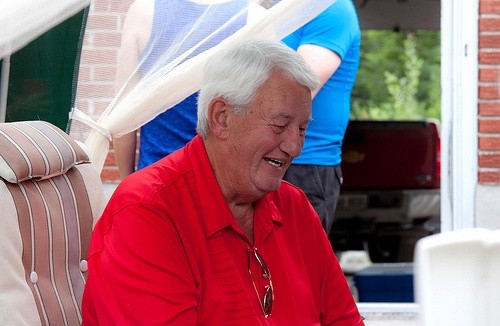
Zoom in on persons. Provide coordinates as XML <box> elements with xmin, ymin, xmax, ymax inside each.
<box><xmin>80</xmin><ymin>36</ymin><xmax>366</xmax><ymax>326</ymax></box>
<box><xmin>246</xmin><ymin>0</ymin><xmax>361</xmax><ymax>239</ymax></box>
<box><xmin>111</xmin><ymin>0</ymin><xmax>251</xmax><ymax>180</ymax></box>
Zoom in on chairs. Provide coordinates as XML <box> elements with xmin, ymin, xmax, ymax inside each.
<box><xmin>0</xmin><ymin>119</ymin><xmax>94</xmax><ymax>326</ymax></box>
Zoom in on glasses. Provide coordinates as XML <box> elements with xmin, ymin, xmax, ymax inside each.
<box><xmin>248</xmin><ymin>246</ymin><xmax>274</xmax><ymax>319</ymax></box>
